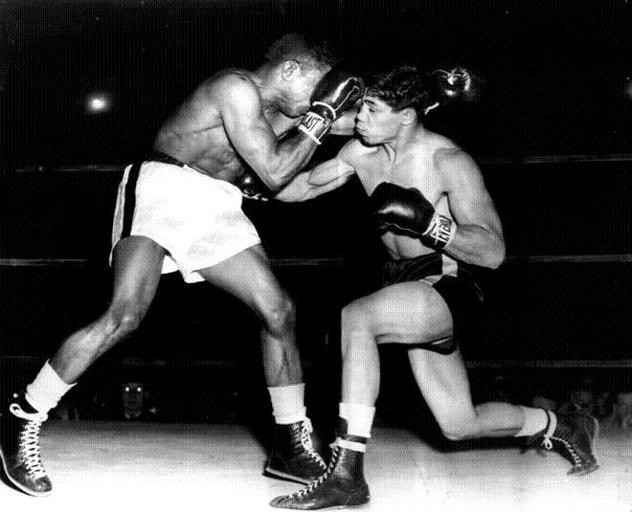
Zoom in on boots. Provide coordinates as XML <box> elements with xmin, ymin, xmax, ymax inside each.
<box><xmin>269</xmin><ymin>433</ymin><xmax>371</xmax><ymax>509</ymax></box>
<box><xmin>264</xmin><ymin>418</ymin><xmax>327</xmax><ymax>484</ymax></box>
<box><xmin>540</xmin><ymin>402</ymin><xmax>601</xmax><ymax>477</ymax></box>
<box><xmin>0</xmin><ymin>390</ymin><xmax>53</xmax><ymax>496</ymax></box>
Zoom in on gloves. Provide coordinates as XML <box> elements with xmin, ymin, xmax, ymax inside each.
<box><xmin>296</xmin><ymin>66</ymin><xmax>365</xmax><ymax>146</ymax></box>
<box><xmin>369</xmin><ymin>182</ymin><xmax>455</xmax><ymax>250</ymax></box>
<box><xmin>428</xmin><ymin>65</ymin><xmax>477</xmax><ymax>103</ymax></box>
<box><xmin>235</xmin><ymin>170</ymin><xmax>274</xmax><ymax>202</ymax></box>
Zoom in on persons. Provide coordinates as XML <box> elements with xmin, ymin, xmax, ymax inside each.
<box><xmin>236</xmin><ymin>62</ymin><xmax>601</xmax><ymax>511</ymax></box>
<box><xmin>111</xmin><ymin>357</ymin><xmax>154</xmax><ymax>422</ymax></box>
<box><xmin>529</xmin><ymin>382</ymin><xmax>632</xmax><ymax>431</ymax></box>
<box><xmin>0</xmin><ymin>31</ymin><xmax>489</xmax><ymax>504</ymax></box>
